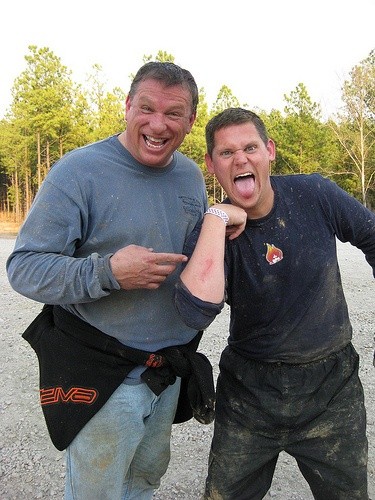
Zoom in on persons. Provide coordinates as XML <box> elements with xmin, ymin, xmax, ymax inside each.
<box><xmin>169</xmin><ymin>106</ymin><xmax>375</xmax><ymax>500</ymax></box>
<box><xmin>4</xmin><ymin>61</ymin><xmax>209</xmax><ymax>500</ymax></box>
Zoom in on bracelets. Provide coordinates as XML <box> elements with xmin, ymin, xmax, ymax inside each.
<box><xmin>202</xmin><ymin>208</ymin><xmax>230</xmax><ymax>227</ymax></box>
